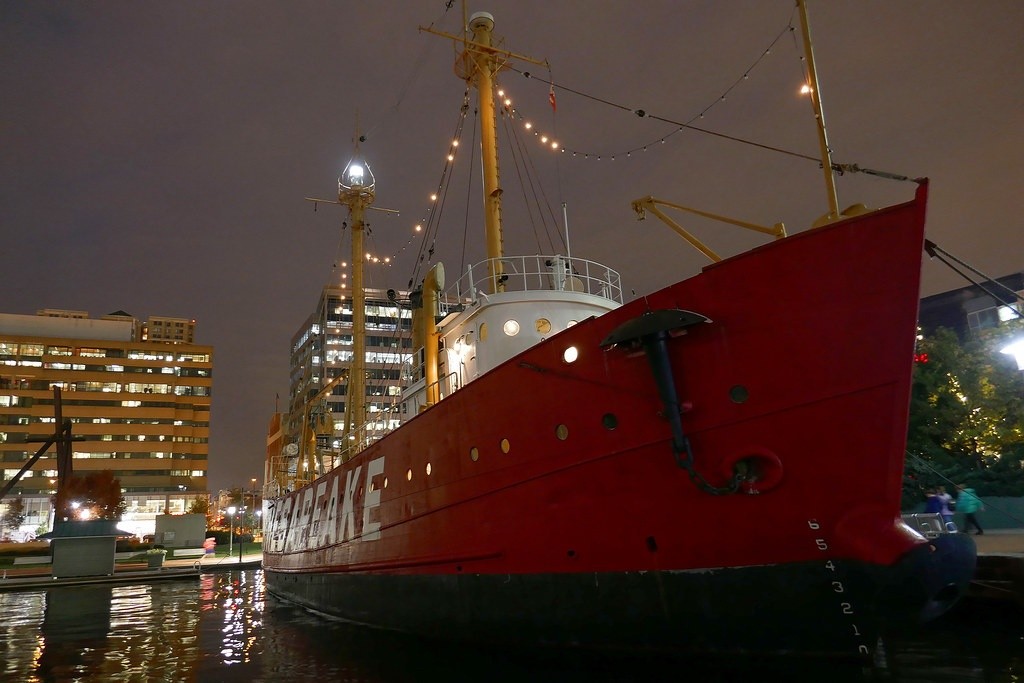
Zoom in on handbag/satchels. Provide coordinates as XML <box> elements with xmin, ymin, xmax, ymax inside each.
<box><xmin>947</xmin><ymin>499</ymin><xmax>957</xmax><ymax>511</ymax></box>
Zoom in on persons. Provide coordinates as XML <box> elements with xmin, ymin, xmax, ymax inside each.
<box><xmin>923</xmin><ymin>484</ymin><xmax>984</xmax><ymax>536</ymax></box>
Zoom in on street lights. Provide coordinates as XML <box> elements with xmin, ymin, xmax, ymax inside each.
<box><xmin>227</xmin><ymin>506</ymin><xmax>237</xmax><ymax>552</ymax></box>
<box><xmin>252</xmin><ymin>478</ymin><xmax>257</xmax><ymax>517</ymax></box>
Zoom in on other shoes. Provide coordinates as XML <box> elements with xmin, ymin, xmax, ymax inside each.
<box><xmin>975</xmin><ymin>531</ymin><xmax>983</xmax><ymax>535</ymax></box>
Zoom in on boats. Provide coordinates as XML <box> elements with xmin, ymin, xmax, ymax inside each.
<box><xmin>257</xmin><ymin>0</ymin><xmax>977</xmax><ymax>657</ymax></box>
